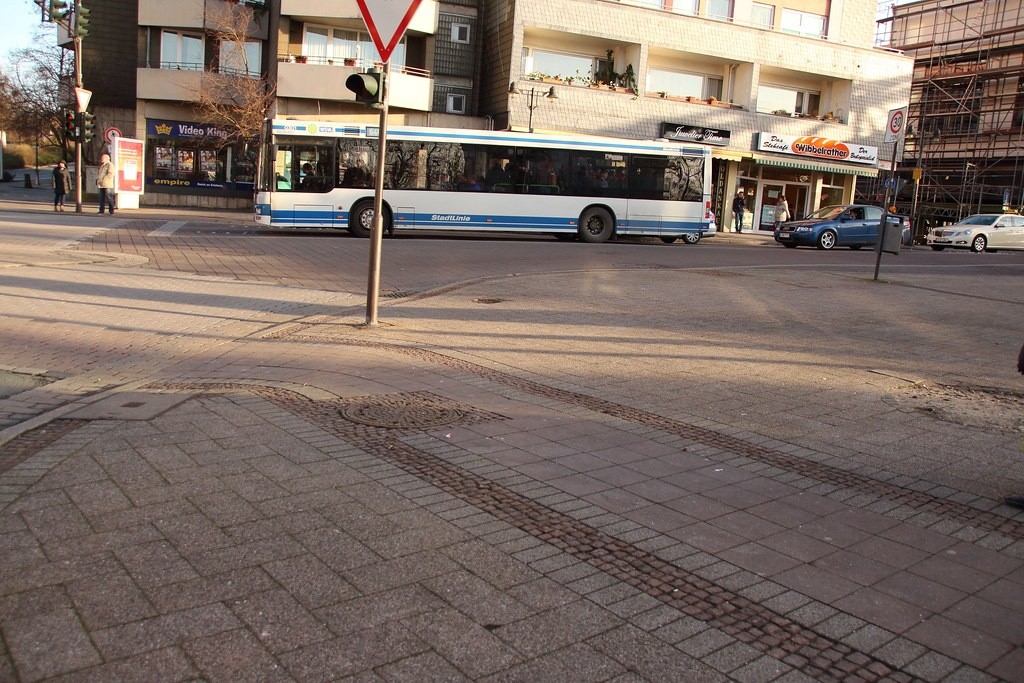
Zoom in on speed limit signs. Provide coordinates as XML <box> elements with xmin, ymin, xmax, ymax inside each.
<box><xmin>885</xmin><ymin>107</ymin><xmax>906</xmax><ymax>144</ymax></box>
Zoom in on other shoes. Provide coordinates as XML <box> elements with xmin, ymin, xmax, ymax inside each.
<box><xmin>736</xmin><ymin>229</ymin><xmax>743</xmax><ymax>234</ymax></box>
<box><xmin>97</xmin><ymin>211</ymin><xmax>115</xmax><ymax>216</ymax></box>
<box><xmin>55</xmin><ymin>205</ymin><xmax>65</xmax><ymax>212</ymax></box>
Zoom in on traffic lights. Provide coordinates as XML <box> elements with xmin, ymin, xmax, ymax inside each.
<box><xmin>76</xmin><ymin>5</ymin><xmax>92</xmax><ymax>37</ymax></box>
<box><xmin>346</xmin><ymin>69</ymin><xmax>385</xmax><ymax>106</ymax></box>
<box><xmin>81</xmin><ymin>111</ymin><xmax>96</xmax><ymax>143</ymax></box>
<box><xmin>50</xmin><ymin>0</ymin><xmax>67</xmax><ymax>22</ymax></box>
<box><xmin>64</xmin><ymin>112</ymin><xmax>77</xmax><ymax>142</ymax></box>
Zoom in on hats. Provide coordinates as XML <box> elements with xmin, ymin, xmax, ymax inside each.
<box><xmin>56</xmin><ymin>159</ymin><xmax>66</xmax><ymax>165</ymax></box>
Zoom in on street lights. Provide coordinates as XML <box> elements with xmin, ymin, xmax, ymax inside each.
<box><xmin>505</xmin><ymin>81</ymin><xmax>558</xmax><ymax>132</ymax></box>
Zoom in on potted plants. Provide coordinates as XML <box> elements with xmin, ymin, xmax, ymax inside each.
<box><xmin>525</xmin><ymin>50</ymin><xmax>641</xmax><ymax>102</ymax></box>
<box><xmin>327</xmin><ymin>59</ymin><xmax>333</xmax><ymax>65</ymax></box>
<box><xmin>344</xmin><ymin>58</ymin><xmax>354</xmax><ymax>67</ymax></box>
<box><xmin>294</xmin><ymin>55</ymin><xmax>308</xmax><ymax>63</ymax></box>
<box><xmin>643</xmin><ymin>92</ymin><xmax>731</xmax><ymax>109</ymax></box>
<box><xmin>773</xmin><ymin>110</ymin><xmax>791</xmax><ymax>118</ymax></box>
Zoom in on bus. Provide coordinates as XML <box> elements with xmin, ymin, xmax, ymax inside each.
<box><xmin>251</xmin><ymin>117</ymin><xmax>712</xmax><ymax>244</ymax></box>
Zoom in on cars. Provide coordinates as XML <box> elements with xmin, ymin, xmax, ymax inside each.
<box><xmin>774</xmin><ymin>204</ymin><xmax>912</xmax><ymax>250</ymax></box>
<box><xmin>926</xmin><ymin>214</ymin><xmax>1023</xmax><ymax>252</ymax></box>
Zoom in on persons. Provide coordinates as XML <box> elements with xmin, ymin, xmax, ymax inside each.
<box><xmin>466</xmin><ymin>168</ymin><xmax>486</xmax><ymax>192</ymax></box>
<box><xmin>300</xmin><ymin>163</ymin><xmax>315</xmax><ymax>187</ymax></box>
<box><xmin>95</xmin><ymin>154</ymin><xmax>115</xmax><ymax>216</ymax></box>
<box><xmin>484</xmin><ymin>160</ymin><xmax>513</xmax><ymax>192</ymax></box>
<box><xmin>357</xmin><ymin>159</ymin><xmax>374</xmax><ymax>187</ymax></box>
<box><xmin>591</xmin><ymin>167</ymin><xmax>627</xmax><ymax>188</ymax></box>
<box><xmin>774</xmin><ymin>196</ymin><xmax>791</xmax><ymax>244</ymax></box>
<box><xmin>158</xmin><ymin>148</ymin><xmax>172</xmax><ymax>160</ymax></box>
<box><xmin>52</xmin><ymin>160</ymin><xmax>72</xmax><ymax>212</ymax></box>
<box><xmin>180</xmin><ymin>150</ymin><xmax>194</xmax><ymax>164</ymax></box>
<box><xmin>889</xmin><ymin>206</ymin><xmax>896</xmax><ymax>214</ymax></box>
<box><xmin>733</xmin><ymin>191</ymin><xmax>745</xmax><ymax>234</ymax></box>
<box><xmin>850</xmin><ymin>208</ymin><xmax>862</xmax><ymax>221</ymax></box>
<box><xmin>204</xmin><ymin>150</ymin><xmax>216</xmax><ymax>163</ymax></box>
